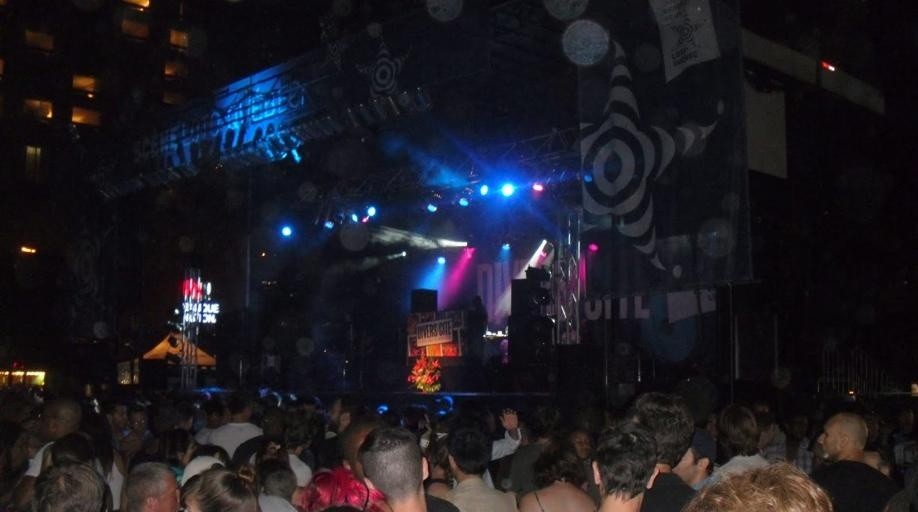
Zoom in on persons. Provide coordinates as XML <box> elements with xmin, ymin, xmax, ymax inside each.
<box><xmin>203</xmin><ymin>326</ymin><xmax>236</xmax><ymax>388</ymax></box>
<box><xmin>468</xmin><ymin>294</ymin><xmax>487</xmax><ymax>362</ymax></box>
<box><xmin>90</xmin><ymin>321</ymin><xmax>120</xmax><ymax>382</ymax></box>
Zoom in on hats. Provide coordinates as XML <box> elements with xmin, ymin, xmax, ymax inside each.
<box><xmin>249</xmin><ymin>449</ymin><xmax>312</xmax><ymax>488</ymax></box>
<box><xmin>180</xmin><ymin>455</ymin><xmax>226</xmax><ymax>489</ymax></box>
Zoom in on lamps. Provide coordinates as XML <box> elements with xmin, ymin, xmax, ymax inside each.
<box><xmin>253</xmin><ymin>71</ymin><xmax>438</xmax><ymax>167</ymax></box>
<box><xmin>305</xmin><ymin>144</ymin><xmax>580</xmax><ymax>238</ymax></box>
